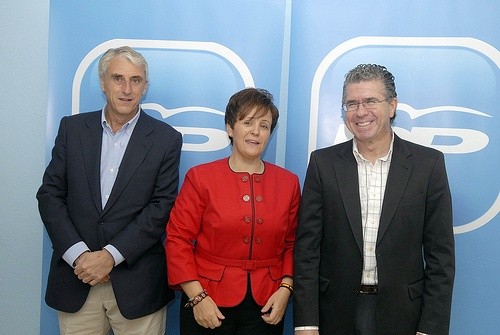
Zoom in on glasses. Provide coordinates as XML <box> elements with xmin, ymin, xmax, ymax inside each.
<box><xmin>341</xmin><ymin>97</ymin><xmax>390</xmax><ymax>111</ymax></box>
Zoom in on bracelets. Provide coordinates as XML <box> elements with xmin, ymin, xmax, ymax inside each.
<box><xmin>184</xmin><ymin>288</ymin><xmax>208</xmax><ymax>309</ymax></box>
<box><xmin>278</xmin><ymin>283</ymin><xmax>294</xmax><ymax>294</ymax></box>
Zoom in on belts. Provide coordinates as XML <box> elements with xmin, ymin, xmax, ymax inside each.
<box><xmin>360</xmin><ymin>285</ymin><xmax>377</xmax><ymax>294</ymax></box>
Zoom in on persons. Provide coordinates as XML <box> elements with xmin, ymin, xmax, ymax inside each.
<box><xmin>35</xmin><ymin>45</ymin><xmax>184</xmax><ymax>335</ymax></box>
<box><xmin>292</xmin><ymin>63</ymin><xmax>457</xmax><ymax>335</ymax></box>
<box><xmin>162</xmin><ymin>87</ymin><xmax>301</xmax><ymax>335</ymax></box>
